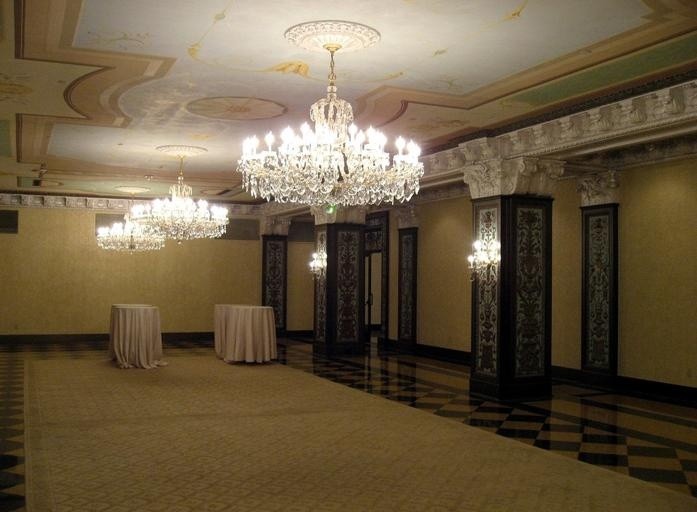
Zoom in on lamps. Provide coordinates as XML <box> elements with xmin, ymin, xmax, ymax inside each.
<box><xmin>236</xmin><ymin>20</ymin><xmax>426</xmax><ymax>208</ymax></box>
<box><xmin>308</xmin><ymin>251</ymin><xmax>327</xmax><ymax>274</ymax></box>
<box><xmin>123</xmin><ymin>143</ymin><xmax>230</xmax><ymax>242</ymax></box>
<box><xmin>95</xmin><ymin>186</ymin><xmax>167</xmax><ymax>252</ymax></box>
<box><xmin>467</xmin><ymin>240</ymin><xmax>501</xmax><ymax>272</ymax></box>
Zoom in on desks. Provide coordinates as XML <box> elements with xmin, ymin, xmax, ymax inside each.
<box><xmin>212</xmin><ymin>303</ymin><xmax>278</xmax><ymax>364</ymax></box>
<box><xmin>107</xmin><ymin>303</ymin><xmax>168</xmax><ymax>370</ymax></box>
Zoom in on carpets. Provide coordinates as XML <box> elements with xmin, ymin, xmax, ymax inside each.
<box><xmin>21</xmin><ymin>352</ymin><xmax>697</xmax><ymax>512</ymax></box>
